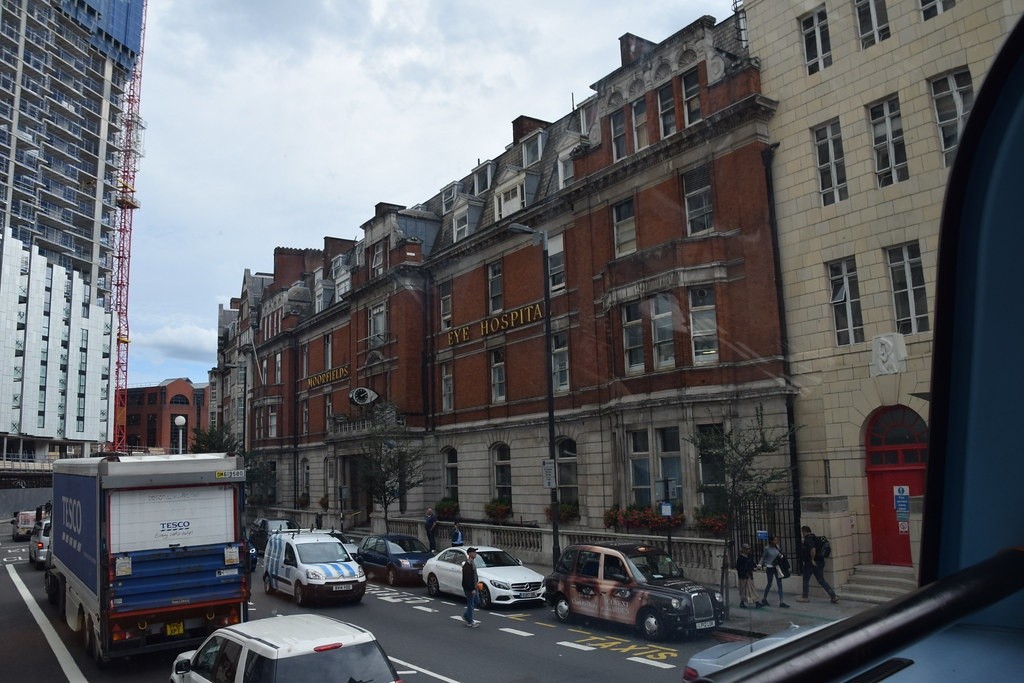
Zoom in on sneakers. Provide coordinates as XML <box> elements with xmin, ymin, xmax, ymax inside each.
<box><xmin>796</xmin><ymin>598</ymin><xmax>809</xmax><ymax>603</ymax></box>
<box><xmin>739</xmin><ymin>601</ymin><xmax>747</xmax><ymax>608</ymax></box>
<box><xmin>831</xmin><ymin>596</ymin><xmax>839</xmax><ymax>603</ymax></box>
<box><xmin>780</xmin><ymin>603</ymin><xmax>790</xmax><ymax>607</ymax></box>
<box><xmin>762</xmin><ymin>599</ymin><xmax>770</xmax><ymax>606</ymax></box>
<box><xmin>471</xmin><ymin>622</ymin><xmax>479</xmax><ymax>628</ymax></box>
<box><xmin>462</xmin><ymin>616</ymin><xmax>472</xmax><ymax>626</ymax></box>
<box><xmin>756</xmin><ymin>601</ymin><xmax>764</xmax><ymax>608</ymax></box>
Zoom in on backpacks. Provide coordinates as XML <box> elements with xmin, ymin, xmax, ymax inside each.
<box><xmin>805</xmin><ymin>536</ymin><xmax>831</xmax><ymax>557</ymax></box>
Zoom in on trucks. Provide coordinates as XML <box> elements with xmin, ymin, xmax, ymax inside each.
<box><xmin>44</xmin><ymin>447</ymin><xmax>253</xmax><ymax>668</ymax></box>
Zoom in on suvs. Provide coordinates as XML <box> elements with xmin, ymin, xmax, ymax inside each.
<box><xmin>166</xmin><ymin>612</ymin><xmax>404</xmax><ymax>683</ymax></box>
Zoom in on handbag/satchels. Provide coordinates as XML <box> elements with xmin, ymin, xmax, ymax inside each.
<box><xmin>776</xmin><ymin>563</ymin><xmax>790</xmax><ymax>579</ymax></box>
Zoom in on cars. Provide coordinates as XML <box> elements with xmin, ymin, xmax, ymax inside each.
<box><xmin>301</xmin><ymin>528</ymin><xmax>358</xmax><ymax>560</ymax></box>
<box><xmin>681</xmin><ymin>625</ymin><xmax>822</xmax><ymax>683</ymax></box>
<box><xmin>422</xmin><ymin>546</ymin><xmax>547</xmax><ymax>610</ymax></box>
<box><xmin>248</xmin><ymin>540</ymin><xmax>258</xmax><ymax>572</ymax></box>
<box><xmin>356</xmin><ymin>535</ymin><xmax>432</xmax><ymax>587</ymax></box>
<box><xmin>543</xmin><ymin>541</ymin><xmax>726</xmax><ymax>639</ymax></box>
<box><xmin>249</xmin><ymin>517</ymin><xmax>302</xmax><ymax>553</ymax></box>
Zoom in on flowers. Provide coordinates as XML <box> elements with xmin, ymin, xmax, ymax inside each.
<box><xmin>544</xmin><ymin>503</ymin><xmax>578</xmax><ymax>523</ymax></box>
<box><xmin>319</xmin><ymin>497</ymin><xmax>328</xmax><ymax>512</ymax></box>
<box><xmin>483</xmin><ymin>500</ymin><xmax>510</xmax><ymax>519</ymax></box>
<box><xmin>696</xmin><ymin>505</ymin><xmax>733</xmax><ymax>534</ymax></box>
<box><xmin>298</xmin><ymin>493</ymin><xmax>310</xmax><ymax>510</ymax></box>
<box><xmin>434</xmin><ymin>498</ymin><xmax>459</xmax><ymax>518</ymax></box>
<box><xmin>603</xmin><ymin>501</ymin><xmax>685</xmax><ymax>530</ymax></box>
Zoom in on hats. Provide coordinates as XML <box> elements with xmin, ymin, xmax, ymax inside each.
<box><xmin>467</xmin><ymin>548</ymin><xmax>478</xmax><ymax>553</ymax></box>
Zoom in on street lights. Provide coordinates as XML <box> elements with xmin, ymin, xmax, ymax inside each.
<box><xmin>509</xmin><ymin>221</ymin><xmax>562</xmax><ymax>574</ymax></box>
<box><xmin>175</xmin><ymin>416</ymin><xmax>186</xmax><ymax>455</ymax></box>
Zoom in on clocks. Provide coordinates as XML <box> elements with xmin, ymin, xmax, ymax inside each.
<box><xmin>355</xmin><ymin>389</ymin><xmax>368</xmax><ymax>404</ymax></box>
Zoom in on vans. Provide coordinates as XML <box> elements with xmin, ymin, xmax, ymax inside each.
<box><xmin>29</xmin><ymin>519</ymin><xmax>51</xmax><ymax>569</ymax></box>
<box><xmin>262</xmin><ymin>531</ymin><xmax>366</xmax><ymax>607</ymax></box>
<box><xmin>11</xmin><ymin>511</ymin><xmax>49</xmax><ymax>542</ymax></box>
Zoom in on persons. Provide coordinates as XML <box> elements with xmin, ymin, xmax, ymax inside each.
<box><xmin>424</xmin><ymin>508</ymin><xmax>437</xmax><ymax>553</ymax></box>
<box><xmin>795</xmin><ymin>526</ymin><xmax>840</xmax><ymax>603</ymax></box>
<box><xmin>757</xmin><ymin>535</ymin><xmax>791</xmax><ymax>609</ymax></box>
<box><xmin>451</xmin><ymin>521</ymin><xmax>464</xmax><ymax>547</ymax></box>
<box><xmin>736</xmin><ymin>543</ymin><xmax>766</xmax><ymax>609</ymax></box>
<box><xmin>461</xmin><ymin>547</ymin><xmax>480</xmax><ymax>628</ymax></box>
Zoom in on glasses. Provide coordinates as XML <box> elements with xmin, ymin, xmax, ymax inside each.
<box><xmin>745</xmin><ymin>547</ymin><xmax>751</xmax><ymax>550</ymax></box>
